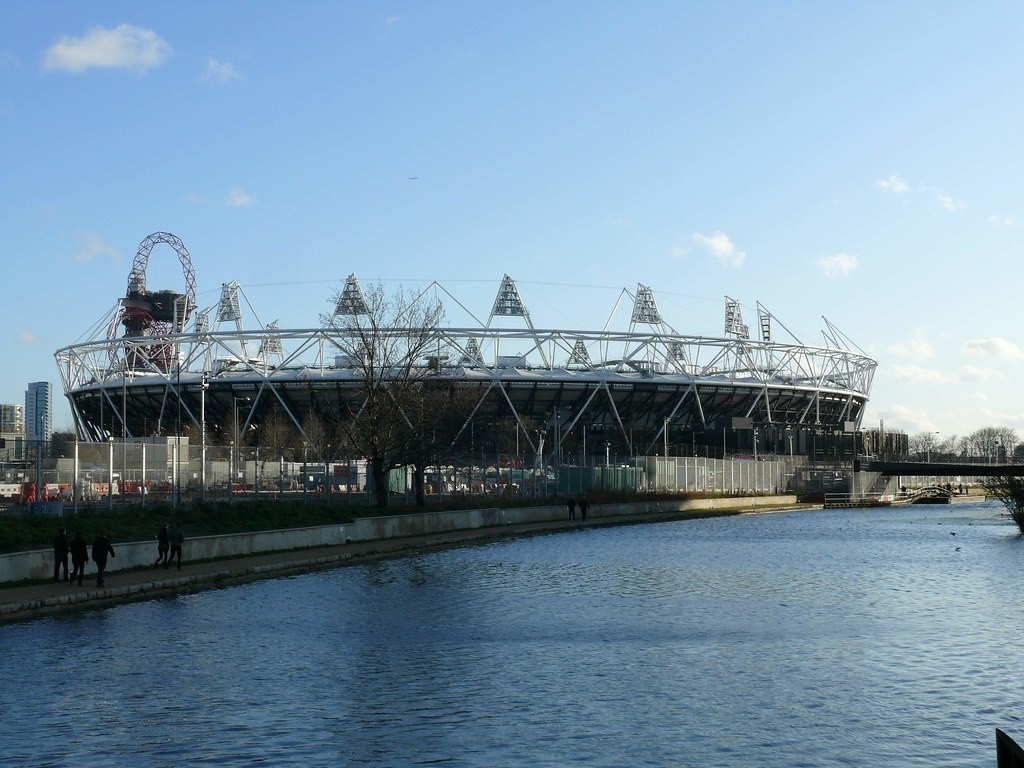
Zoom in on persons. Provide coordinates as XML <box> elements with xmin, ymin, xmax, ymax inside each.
<box><xmin>901</xmin><ymin>485</ymin><xmax>906</xmax><ymax>499</ymax></box>
<box><xmin>54</xmin><ymin>527</ymin><xmax>71</xmax><ymax>585</ymax></box>
<box><xmin>579</xmin><ymin>496</ymin><xmax>590</xmax><ymax>522</ymax></box>
<box><xmin>164</xmin><ymin>522</ymin><xmax>185</xmax><ymax>571</ymax></box>
<box><xmin>959</xmin><ymin>484</ymin><xmax>963</xmax><ymax>494</ymax></box>
<box><xmin>69</xmin><ymin>530</ymin><xmax>89</xmax><ymax>586</ymax></box>
<box><xmin>154</xmin><ymin>523</ymin><xmax>169</xmax><ymax>569</ymax></box>
<box><xmin>92</xmin><ymin>532</ymin><xmax>115</xmax><ymax>586</ymax></box>
<box><xmin>567</xmin><ymin>496</ymin><xmax>577</xmax><ymax>520</ymax></box>
<box><xmin>952</xmin><ymin>488</ymin><xmax>959</xmax><ymax>493</ymax></box>
